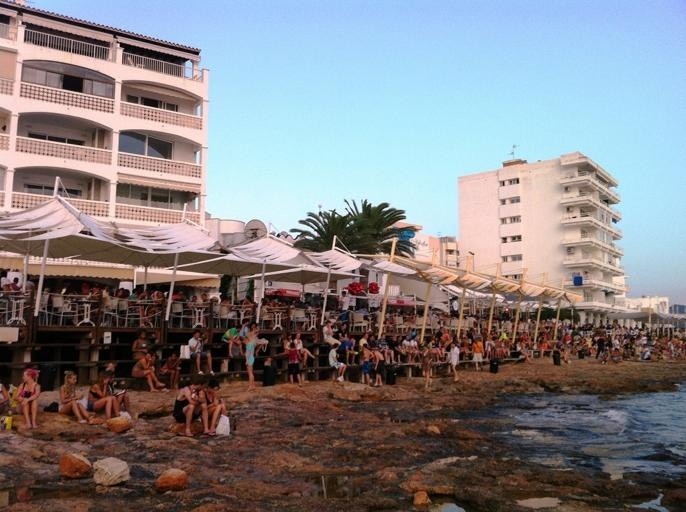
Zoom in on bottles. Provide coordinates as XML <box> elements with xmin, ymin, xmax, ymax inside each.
<box><xmin>5</xmin><ymin>411</ymin><xmax>13</xmax><ymax>429</ymax></box>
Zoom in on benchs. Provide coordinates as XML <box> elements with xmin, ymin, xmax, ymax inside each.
<box><xmin>1</xmin><ymin>338</ymin><xmax>489</xmax><ymax>385</ymax></box>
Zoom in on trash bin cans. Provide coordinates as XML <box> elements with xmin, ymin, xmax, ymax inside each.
<box><xmin>552</xmin><ymin>350</ymin><xmax>560</xmax><ymax>365</ymax></box>
<box><xmin>386</xmin><ymin>363</ymin><xmax>397</xmax><ymax>385</ymax></box>
<box><xmin>490</xmin><ymin>360</ymin><xmax>498</xmax><ymax>373</ymax></box>
<box><xmin>578</xmin><ymin>350</ymin><xmax>584</xmax><ymax>359</ymax></box>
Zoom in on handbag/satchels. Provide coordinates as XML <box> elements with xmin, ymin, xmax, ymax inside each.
<box><xmin>215</xmin><ymin>413</ymin><xmax>232</xmax><ymax>436</ymax></box>
